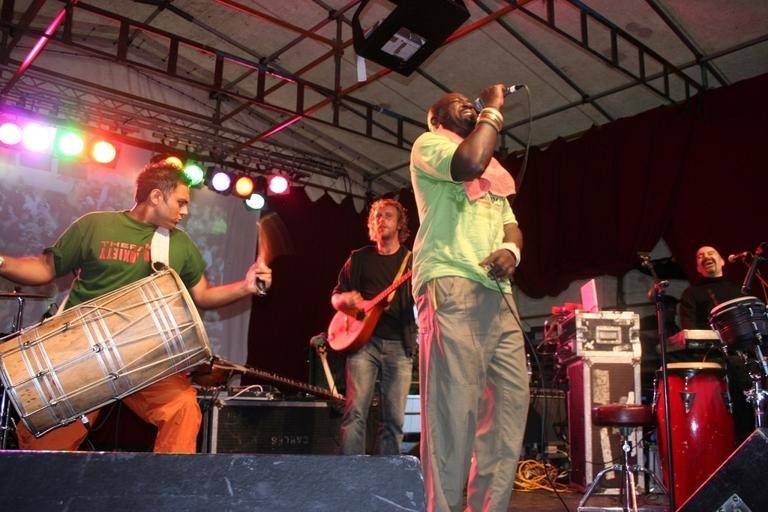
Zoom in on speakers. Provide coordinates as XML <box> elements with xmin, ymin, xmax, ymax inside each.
<box><xmin>0</xmin><ymin>451</ymin><xmax>426</xmax><ymax>512</ymax></box>
<box><xmin>674</xmin><ymin>427</ymin><xmax>768</xmax><ymax>512</ymax></box>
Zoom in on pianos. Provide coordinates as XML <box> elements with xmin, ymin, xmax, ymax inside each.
<box><xmin>657</xmin><ymin>328</ymin><xmax>723</xmax><ymax>354</ymax></box>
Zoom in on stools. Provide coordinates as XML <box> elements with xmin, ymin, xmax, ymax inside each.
<box><xmin>578</xmin><ymin>405</ymin><xmax>670</xmax><ymax>512</ymax></box>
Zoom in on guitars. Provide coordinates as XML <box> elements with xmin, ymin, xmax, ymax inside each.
<box><xmin>327</xmin><ymin>270</ymin><xmax>413</xmax><ymax>353</ymax></box>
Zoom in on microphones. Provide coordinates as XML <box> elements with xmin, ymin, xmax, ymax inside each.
<box><xmin>474</xmin><ymin>85</ymin><xmax>520</xmax><ymax>114</ymax></box>
<box><xmin>728</xmin><ymin>251</ymin><xmax>748</xmax><ymax>263</ymax></box>
<box><xmin>741</xmin><ymin>259</ymin><xmax>759</xmax><ymax>296</ymax></box>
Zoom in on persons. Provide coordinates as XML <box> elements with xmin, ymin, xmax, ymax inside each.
<box><xmin>330</xmin><ymin>196</ymin><xmax>416</xmax><ymax>456</ymax></box>
<box><xmin>679</xmin><ymin>244</ymin><xmax>742</xmax><ymax>331</ymax></box>
<box><xmin>0</xmin><ymin>161</ymin><xmax>274</xmax><ymax>453</ymax></box>
<box><xmin>406</xmin><ymin>81</ymin><xmax>532</xmax><ymax>511</ymax></box>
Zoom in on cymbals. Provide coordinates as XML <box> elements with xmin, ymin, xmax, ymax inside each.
<box><xmin>3</xmin><ymin>291</ymin><xmax>55</xmax><ymax>300</ymax></box>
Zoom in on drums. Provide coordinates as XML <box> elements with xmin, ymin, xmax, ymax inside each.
<box><xmin>709</xmin><ymin>296</ymin><xmax>768</xmax><ymax>343</ymax></box>
<box><xmin>656</xmin><ymin>361</ymin><xmax>736</xmax><ymax>512</ymax></box>
<box><xmin>1</xmin><ymin>269</ymin><xmax>213</xmax><ymax>437</ymax></box>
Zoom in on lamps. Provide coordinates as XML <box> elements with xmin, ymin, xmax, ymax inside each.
<box><xmin>267</xmin><ymin>174</ymin><xmax>290</xmax><ymax>196</ymax></box>
<box><xmin>52</xmin><ymin>125</ymin><xmax>86</xmax><ymax>156</ymax></box>
<box><xmin>183</xmin><ymin>162</ymin><xmax>206</xmax><ymax>190</ymax></box>
<box><xmin>23</xmin><ymin>120</ymin><xmax>52</xmax><ymax>154</ymax></box>
<box><xmin>158</xmin><ymin>152</ymin><xmax>183</xmax><ymax>172</ymax></box>
<box><xmin>242</xmin><ymin>192</ymin><xmax>271</xmax><ymax>212</ymax></box>
<box><xmin>1</xmin><ymin>117</ymin><xmax>23</xmax><ymax>150</ymax></box>
<box><xmin>231</xmin><ymin>173</ymin><xmax>257</xmax><ymax>199</ymax></box>
<box><xmin>206</xmin><ymin>167</ymin><xmax>231</xmax><ymax>194</ymax></box>
<box><xmin>91</xmin><ymin>135</ymin><xmax>121</xmax><ymax>169</ymax></box>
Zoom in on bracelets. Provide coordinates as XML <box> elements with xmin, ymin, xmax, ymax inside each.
<box><xmin>495</xmin><ymin>242</ymin><xmax>522</xmax><ymax>268</ymax></box>
<box><xmin>475</xmin><ymin>106</ymin><xmax>506</xmax><ymax>134</ymax></box>
